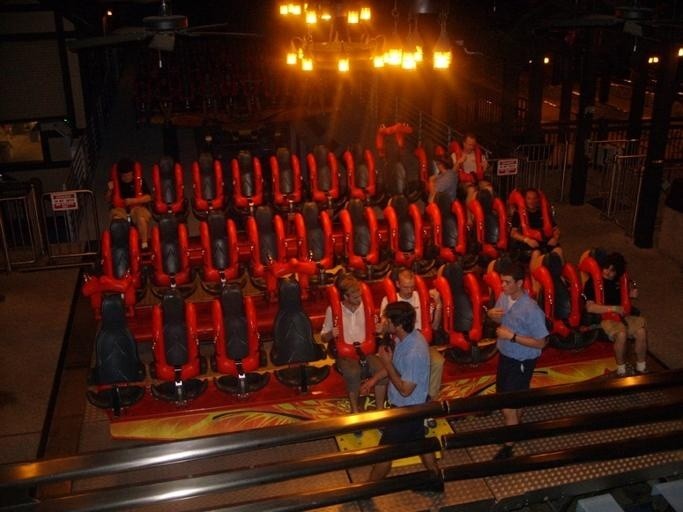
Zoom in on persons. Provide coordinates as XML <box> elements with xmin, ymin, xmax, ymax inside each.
<box><xmin>379</xmin><ymin>270</ymin><xmax>445</xmax><ymax>431</ymax></box>
<box><xmin>104</xmin><ymin>160</ymin><xmax>154</xmax><ymax>250</ymax></box>
<box><xmin>357</xmin><ymin>301</ymin><xmax>442</xmax><ymax>499</ymax></box>
<box><xmin>582</xmin><ymin>252</ymin><xmax>649</xmax><ymax>379</ymax></box>
<box><xmin>510</xmin><ymin>187</ymin><xmax>565</xmax><ymax>267</ymax></box>
<box><xmin>319</xmin><ymin>277</ymin><xmax>389</xmax><ymax>413</ymax></box>
<box><xmin>427</xmin><ymin>154</ymin><xmax>458</xmax><ymax>203</ymax></box>
<box><xmin>487</xmin><ymin>261</ymin><xmax>550</xmax><ymax>460</ymax></box>
<box><xmin>449</xmin><ymin>133</ymin><xmax>493</xmax><ymax>226</ymax></box>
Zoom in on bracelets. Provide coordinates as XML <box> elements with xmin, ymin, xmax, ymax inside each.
<box><xmin>608</xmin><ymin>305</ymin><xmax>612</xmax><ymax>313</ymax></box>
<box><xmin>510</xmin><ymin>334</ymin><xmax>516</xmax><ymax>343</ymax></box>
<box><xmin>611</xmin><ymin>304</ymin><xmax>617</xmax><ymax>312</ymax></box>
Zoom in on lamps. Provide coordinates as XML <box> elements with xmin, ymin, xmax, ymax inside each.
<box><xmin>272</xmin><ymin>1</ymin><xmax>458</xmax><ymax>76</ymax></box>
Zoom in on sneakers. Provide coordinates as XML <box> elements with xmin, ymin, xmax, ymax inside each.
<box><xmin>492</xmin><ymin>447</ymin><xmax>514</xmax><ymax>461</ymax></box>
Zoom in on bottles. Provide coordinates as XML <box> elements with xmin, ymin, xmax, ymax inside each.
<box><xmin>308</xmin><ymin>249</ymin><xmax>318</xmax><ymax>283</ymax></box>
<box><xmin>265</xmin><ymin>253</ymin><xmax>277</xmax><ymax>265</ymax></box>
<box><xmin>630</xmin><ymin>280</ymin><xmax>638</xmax><ymax>300</ymax></box>
<box><xmin>551</xmin><ymin>205</ymin><xmax>557</xmax><ymax>216</ymax></box>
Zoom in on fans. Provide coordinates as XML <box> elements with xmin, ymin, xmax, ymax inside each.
<box><xmin>66</xmin><ymin>1</ymin><xmax>264</xmax><ymax>67</ymax></box>
